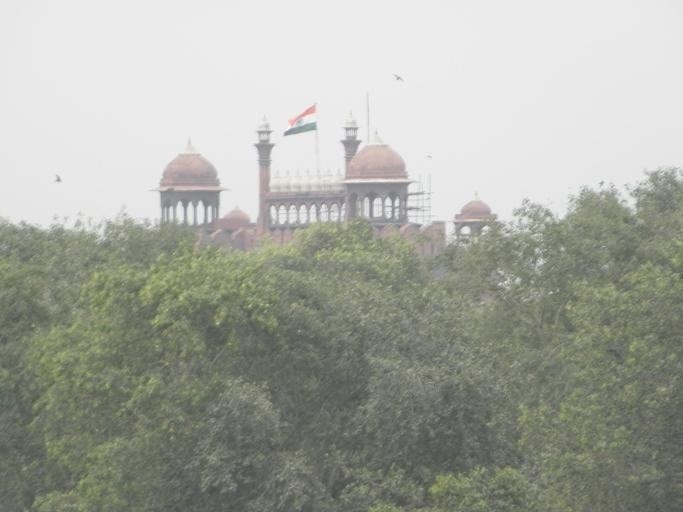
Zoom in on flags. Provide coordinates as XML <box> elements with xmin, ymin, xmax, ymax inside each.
<box><xmin>283</xmin><ymin>102</ymin><xmax>316</xmax><ymax>137</ymax></box>
